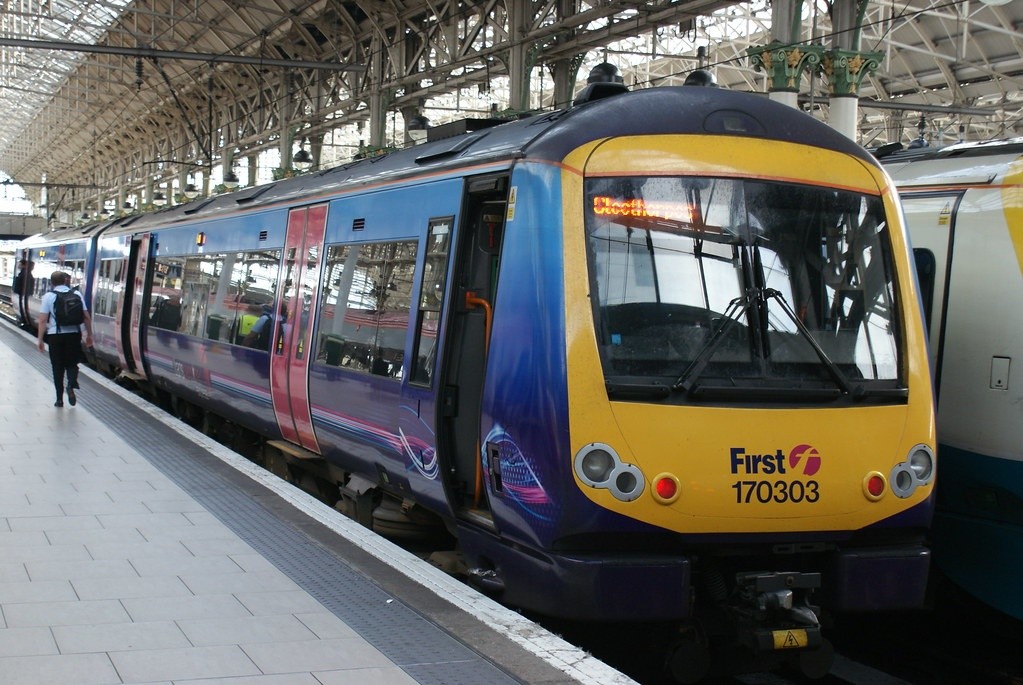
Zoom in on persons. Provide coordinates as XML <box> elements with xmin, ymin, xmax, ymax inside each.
<box><xmin>38</xmin><ymin>271</ymin><xmax>93</xmax><ymax>407</ymax></box>
<box><xmin>153</xmin><ymin>270</ymin><xmax>431</xmax><ymax>386</ymax></box>
<box><xmin>12</xmin><ymin>260</ymin><xmax>28</xmax><ymax>326</ymax></box>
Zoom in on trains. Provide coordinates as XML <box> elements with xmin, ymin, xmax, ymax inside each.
<box><xmin>0</xmin><ymin>68</ymin><xmax>939</xmax><ymax>673</ymax></box>
<box><xmin>590</xmin><ymin>108</ymin><xmax>1023</xmax><ymax>465</ymax></box>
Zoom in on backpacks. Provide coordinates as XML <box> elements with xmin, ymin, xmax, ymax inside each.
<box><xmin>49</xmin><ymin>287</ymin><xmax>82</xmax><ymax>327</ymax></box>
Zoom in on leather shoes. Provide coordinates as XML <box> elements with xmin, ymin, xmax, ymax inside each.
<box><xmin>54</xmin><ymin>400</ymin><xmax>63</xmax><ymax>407</ymax></box>
<box><xmin>66</xmin><ymin>385</ymin><xmax>77</xmax><ymax>407</ymax></box>
